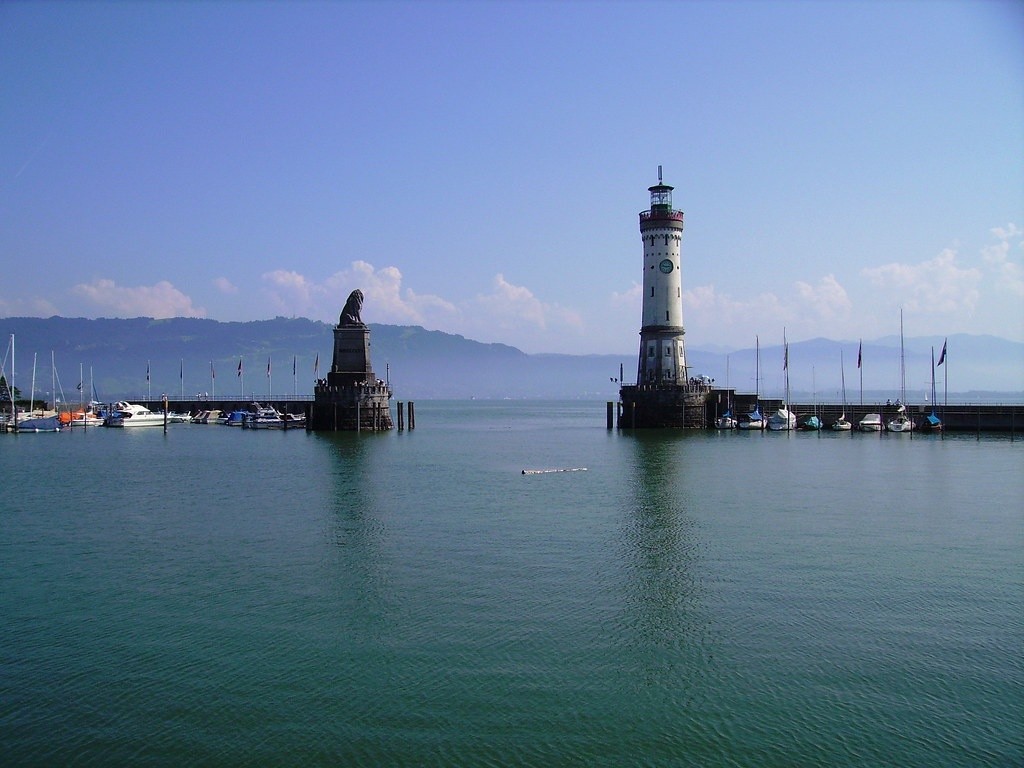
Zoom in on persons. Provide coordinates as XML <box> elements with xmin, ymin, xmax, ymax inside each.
<box><xmin>314</xmin><ymin>378</ymin><xmax>327</xmax><ymax>386</ymax></box>
<box><xmin>198</xmin><ymin>392</ymin><xmax>208</xmax><ymax>401</ymax></box>
<box><xmin>375</xmin><ymin>379</ymin><xmax>385</xmax><ymax>392</ymax></box>
<box><xmin>353</xmin><ymin>379</ymin><xmax>368</xmax><ymax>390</ymax></box>
<box><xmin>161</xmin><ymin>393</ymin><xmax>164</xmax><ymax>400</ymax></box>
<box><xmin>886</xmin><ymin>399</ymin><xmax>901</xmax><ymax>406</ymax></box>
<box><xmin>690</xmin><ymin>377</ymin><xmax>704</xmax><ymax>385</ymax></box>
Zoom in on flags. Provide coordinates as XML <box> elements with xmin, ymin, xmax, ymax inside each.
<box><xmin>314</xmin><ymin>355</ymin><xmax>319</xmax><ymax>373</ymax></box>
<box><xmin>294</xmin><ymin>357</ymin><xmax>295</xmax><ymax>375</ymax></box>
<box><xmin>784</xmin><ymin>347</ymin><xmax>788</xmax><ymax>370</ymax></box>
<box><xmin>857</xmin><ymin>343</ymin><xmax>861</xmax><ymax>367</ymax></box>
<box><xmin>147</xmin><ymin>365</ymin><xmax>150</xmax><ymax>380</ymax></box>
<box><xmin>181</xmin><ymin>361</ymin><xmax>182</xmax><ymax>378</ymax></box>
<box><xmin>238</xmin><ymin>359</ymin><xmax>241</xmax><ymax>377</ymax></box>
<box><xmin>267</xmin><ymin>358</ymin><xmax>270</xmax><ymax>377</ymax></box>
<box><xmin>936</xmin><ymin>341</ymin><xmax>947</xmax><ymax>366</ymax></box>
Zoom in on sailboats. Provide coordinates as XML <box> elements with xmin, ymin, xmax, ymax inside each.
<box><xmin>716</xmin><ymin>356</ymin><xmax>738</xmax><ymax>429</ymax></box>
<box><xmin>919</xmin><ymin>346</ymin><xmax>945</xmax><ymax>432</ymax></box>
<box><xmin>887</xmin><ymin>309</ymin><xmax>916</xmax><ymax>432</ymax></box>
<box><xmin>801</xmin><ymin>367</ymin><xmax>823</xmax><ymax>431</ymax></box>
<box><xmin>735</xmin><ymin>335</ymin><xmax>767</xmax><ymax>430</ymax></box>
<box><xmin>832</xmin><ymin>349</ymin><xmax>851</xmax><ymax>430</ymax></box>
<box><xmin>0</xmin><ymin>334</ymin><xmax>106</xmax><ymax>434</ymax></box>
<box><xmin>769</xmin><ymin>327</ymin><xmax>796</xmax><ymax>431</ymax></box>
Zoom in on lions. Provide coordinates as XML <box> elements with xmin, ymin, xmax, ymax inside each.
<box><xmin>339</xmin><ymin>289</ymin><xmax>366</xmax><ymax>324</ymax></box>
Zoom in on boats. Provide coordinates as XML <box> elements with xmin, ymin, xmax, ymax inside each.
<box><xmin>169</xmin><ymin>405</ymin><xmax>308</xmax><ymax>431</ymax></box>
<box><xmin>110</xmin><ymin>404</ymin><xmax>173</xmax><ymax>427</ymax></box>
<box><xmin>859</xmin><ymin>414</ymin><xmax>886</xmax><ymax>432</ymax></box>
